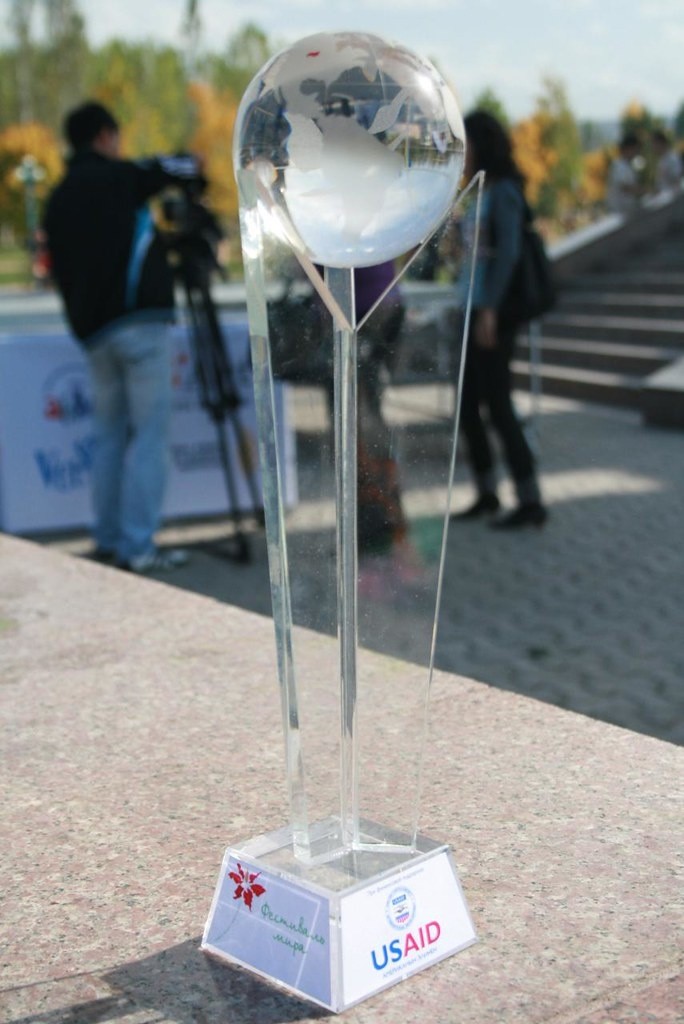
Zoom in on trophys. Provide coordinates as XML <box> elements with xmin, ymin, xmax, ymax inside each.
<box><xmin>192</xmin><ymin>28</ymin><xmax>479</xmax><ymax>1019</ymax></box>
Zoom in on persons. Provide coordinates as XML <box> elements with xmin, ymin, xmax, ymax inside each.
<box><xmin>280</xmin><ymin>255</ymin><xmax>409</xmax><ymax>556</ymax></box>
<box><xmin>603</xmin><ymin>135</ymin><xmax>646</xmax><ymax>213</ymax></box>
<box><xmin>41</xmin><ymin>103</ymin><xmax>202</xmax><ymax>577</ymax></box>
<box><xmin>648</xmin><ymin>128</ymin><xmax>683</xmax><ymax>194</ymax></box>
<box><xmin>437</xmin><ymin>109</ymin><xmax>552</xmax><ymax>533</ymax></box>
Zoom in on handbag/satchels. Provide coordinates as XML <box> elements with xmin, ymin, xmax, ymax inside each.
<box><xmin>487</xmin><ymin>177</ymin><xmax>557</xmax><ymax>332</ymax></box>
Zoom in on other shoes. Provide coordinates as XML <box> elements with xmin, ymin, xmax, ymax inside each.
<box><xmin>124</xmin><ymin>552</ymin><xmax>186</xmax><ymax>570</ymax></box>
<box><xmin>452</xmin><ymin>494</ymin><xmax>501</xmax><ymax>522</ymax></box>
<box><xmin>492</xmin><ymin>503</ymin><xmax>547</xmax><ymax>530</ymax></box>
